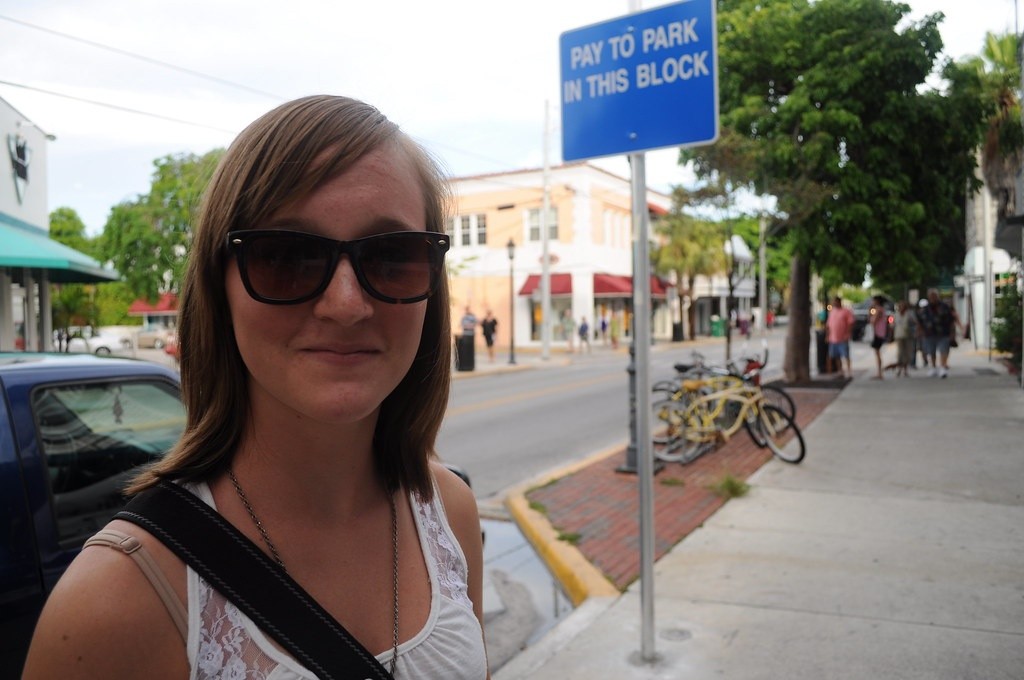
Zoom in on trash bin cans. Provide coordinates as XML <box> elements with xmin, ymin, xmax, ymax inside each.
<box><xmin>454</xmin><ymin>335</ymin><xmax>475</xmax><ymax>371</ymax></box>
<box><xmin>672</xmin><ymin>322</ymin><xmax>684</xmax><ymax>342</ymax></box>
<box><xmin>815</xmin><ymin>329</ymin><xmax>838</xmax><ymax>373</ymax></box>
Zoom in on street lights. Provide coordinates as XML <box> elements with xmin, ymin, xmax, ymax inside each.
<box><xmin>506</xmin><ymin>239</ymin><xmax>530</xmax><ymax>371</ymax></box>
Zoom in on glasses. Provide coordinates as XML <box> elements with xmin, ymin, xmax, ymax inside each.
<box><xmin>226</xmin><ymin>230</ymin><xmax>450</xmax><ymax>305</ymax></box>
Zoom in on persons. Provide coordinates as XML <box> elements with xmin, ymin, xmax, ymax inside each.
<box><xmin>481</xmin><ymin>311</ymin><xmax>497</xmax><ymax>361</ymax></box>
<box><xmin>600</xmin><ymin>313</ymin><xmax>619</xmax><ymax>347</ymax></box>
<box><xmin>461</xmin><ymin>308</ymin><xmax>476</xmax><ymax>331</ymax></box>
<box><xmin>890</xmin><ymin>288</ymin><xmax>962</xmax><ymax>378</ymax></box>
<box><xmin>824</xmin><ymin>296</ymin><xmax>854</xmax><ymax>378</ymax></box>
<box><xmin>744</xmin><ymin>314</ymin><xmax>752</xmax><ymax>338</ymax></box>
<box><xmin>18</xmin><ymin>94</ymin><xmax>491</xmax><ymax>680</ymax></box>
<box><xmin>871</xmin><ymin>296</ymin><xmax>887</xmax><ymax>380</ymax></box>
<box><xmin>560</xmin><ymin>309</ymin><xmax>591</xmax><ymax>351</ymax></box>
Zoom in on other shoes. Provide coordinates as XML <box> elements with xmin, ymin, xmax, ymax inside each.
<box><xmin>938</xmin><ymin>366</ymin><xmax>947</xmax><ymax>380</ymax></box>
<box><xmin>926</xmin><ymin>367</ymin><xmax>938</xmax><ymax>378</ymax></box>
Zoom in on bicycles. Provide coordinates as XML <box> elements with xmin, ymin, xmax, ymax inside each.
<box><xmin>648</xmin><ymin>345</ymin><xmax>811</xmax><ymax>466</ymax></box>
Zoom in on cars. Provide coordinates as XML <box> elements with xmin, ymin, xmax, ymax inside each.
<box><xmin>56</xmin><ymin>326</ymin><xmax>121</xmax><ymax>354</ymax></box>
<box><xmin>846</xmin><ymin>299</ymin><xmax>910</xmax><ymax>340</ymax></box>
<box><xmin>2</xmin><ymin>353</ymin><xmax>486</xmax><ymax>678</ymax></box>
<box><xmin>121</xmin><ymin>323</ymin><xmax>174</xmax><ymax>351</ymax></box>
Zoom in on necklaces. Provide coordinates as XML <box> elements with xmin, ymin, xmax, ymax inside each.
<box><xmin>224</xmin><ymin>468</ymin><xmax>398</xmax><ymax>675</ymax></box>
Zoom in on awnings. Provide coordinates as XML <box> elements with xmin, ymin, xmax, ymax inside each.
<box><xmin>518</xmin><ymin>272</ymin><xmax>667</xmax><ymax>299</ymax></box>
<box><xmin>0</xmin><ymin>221</ymin><xmax>121</xmax><ymax>284</ymax></box>
